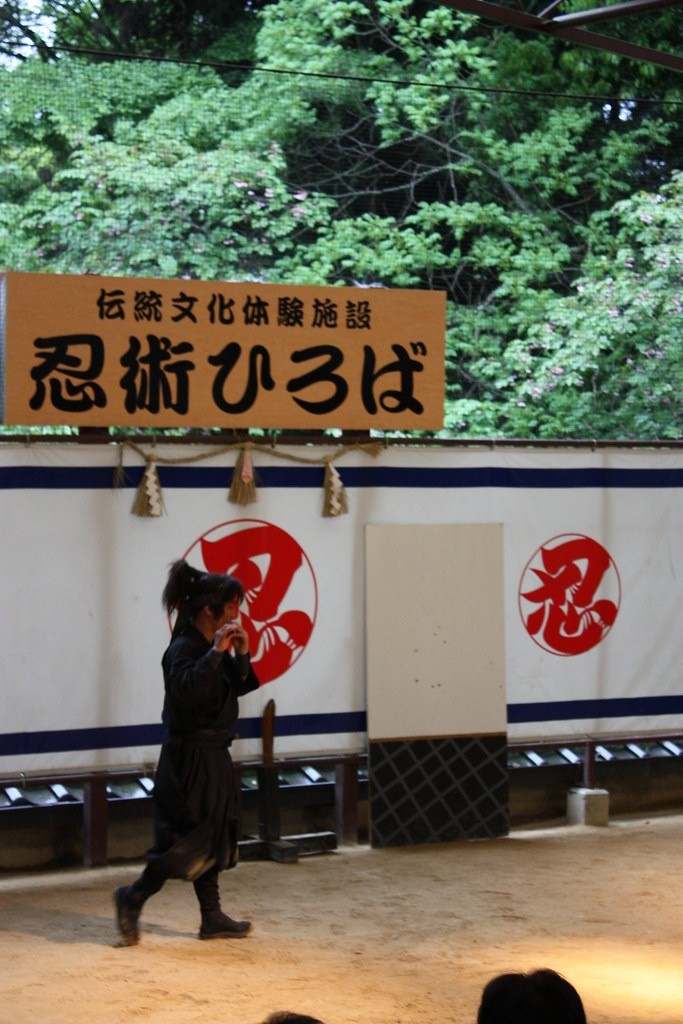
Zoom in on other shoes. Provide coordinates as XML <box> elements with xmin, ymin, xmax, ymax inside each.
<box><xmin>199</xmin><ymin>912</ymin><xmax>252</xmax><ymax>938</ymax></box>
<box><xmin>114</xmin><ymin>883</ymin><xmax>147</xmax><ymax>945</ymax></box>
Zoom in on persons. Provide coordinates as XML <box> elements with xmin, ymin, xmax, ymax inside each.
<box><xmin>115</xmin><ymin>559</ymin><xmax>261</xmax><ymax>945</ymax></box>
<box><xmin>478</xmin><ymin>970</ymin><xmax>587</xmax><ymax>1024</ymax></box>
